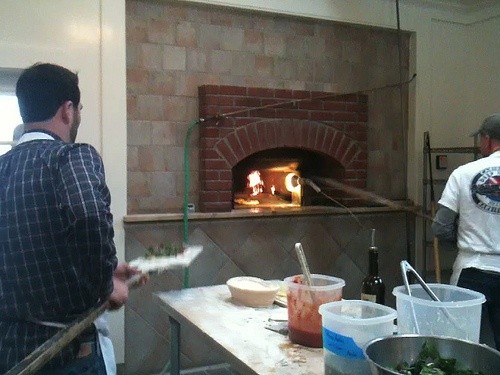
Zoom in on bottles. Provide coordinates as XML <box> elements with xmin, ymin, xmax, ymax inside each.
<box><xmin>361</xmin><ymin>228</ymin><xmax>384</xmax><ymax>305</ymax></box>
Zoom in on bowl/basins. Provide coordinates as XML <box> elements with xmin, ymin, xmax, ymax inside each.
<box><xmin>364</xmin><ymin>333</ymin><xmax>498</xmax><ymax>375</ymax></box>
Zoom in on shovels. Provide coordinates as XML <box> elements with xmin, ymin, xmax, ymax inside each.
<box><xmin>2</xmin><ymin>245</ymin><xmax>203</xmax><ymax>375</ymax></box>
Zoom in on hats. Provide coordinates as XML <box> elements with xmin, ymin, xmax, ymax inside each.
<box><xmin>469</xmin><ymin>114</ymin><xmax>500</xmax><ymax>139</ymax></box>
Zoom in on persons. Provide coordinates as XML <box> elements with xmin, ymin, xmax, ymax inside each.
<box><xmin>431</xmin><ymin>114</ymin><xmax>500</xmax><ymax>352</ymax></box>
<box><xmin>0</xmin><ymin>62</ymin><xmax>149</xmax><ymax>373</ymax></box>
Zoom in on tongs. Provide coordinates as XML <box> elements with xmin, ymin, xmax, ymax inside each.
<box><xmin>399</xmin><ymin>259</ymin><xmax>476</xmax><ymax>333</ymax></box>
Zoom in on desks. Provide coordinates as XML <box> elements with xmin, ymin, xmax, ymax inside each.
<box><xmin>152</xmin><ymin>279</ymin><xmax>497</xmax><ymax>374</ymax></box>
